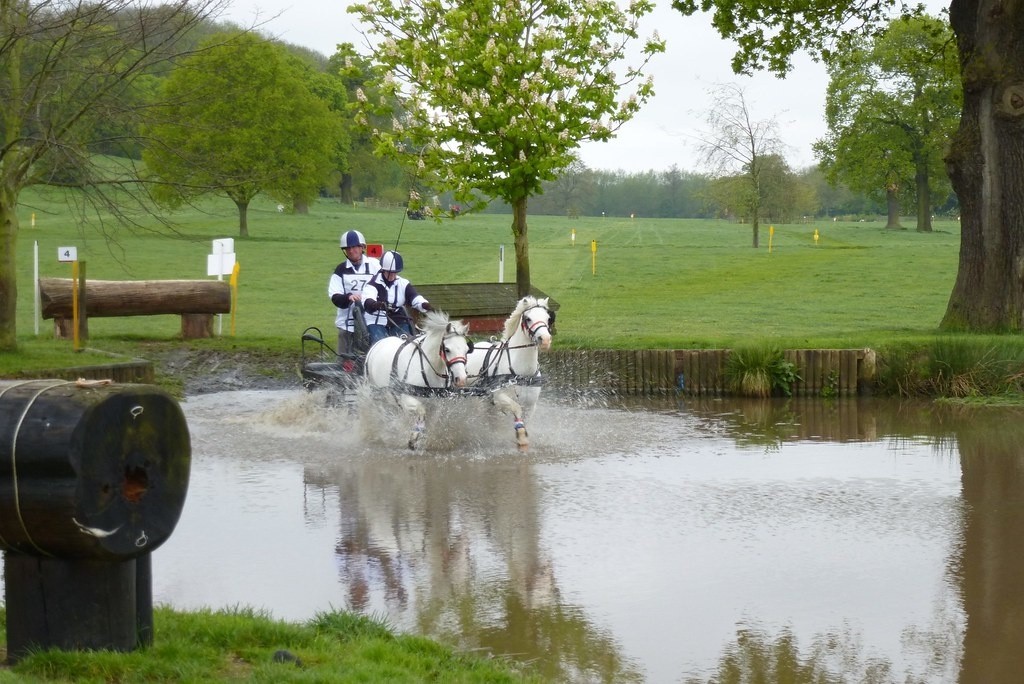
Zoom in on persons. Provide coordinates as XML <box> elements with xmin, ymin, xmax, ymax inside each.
<box><xmin>361</xmin><ymin>251</ymin><xmax>432</xmax><ymax>346</ymax></box>
<box><xmin>328</xmin><ymin>230</ymin><xmax>381</xmax><ymax>362</ymax></box>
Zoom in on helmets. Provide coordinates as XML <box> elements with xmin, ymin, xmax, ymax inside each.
<box><xmin>340</xmin><ymin>230</ymin><xmax>366</xmax><ymax>249</ymax></box>
<box><xmin>380</xmin><ymin>250</ymin><xmax>403</xmax><ymax>272</ymax></box>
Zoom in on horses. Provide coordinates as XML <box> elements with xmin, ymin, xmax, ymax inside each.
<box><xmin>361</xmin><ymin>308</ymin><xmax>470</xmax><ymax>451</ymax></box>
<box><xmin>462</xmin><ymin>295</ymin><xmax>553</xmax><ymax>455</ymax></box>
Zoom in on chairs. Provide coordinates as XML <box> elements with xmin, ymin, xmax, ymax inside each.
<box><xmin>352</xmin><ymin>299</ymin><xmax>415</xmax><ymax>352</ymax></box>
<box><xmin>302</xmin><ymin>327</ymin><xmax>341</xmax><ymax>372</ymax></box>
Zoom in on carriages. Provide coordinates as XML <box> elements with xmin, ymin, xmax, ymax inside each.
<box><xmin>301</xmin><ymin>296</ymin><xmax>556</xmax><ymax>454</ymax></box>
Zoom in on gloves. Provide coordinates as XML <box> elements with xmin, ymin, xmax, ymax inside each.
<box><xmin>423</xmin><ymin>305</ymin><xmax>435</xmax><ymax>312</ymax></box>
<box><xmin>371</xmin><ymin>301</ymin><xmax>387</xmax><ymax>311</ymax></box>
